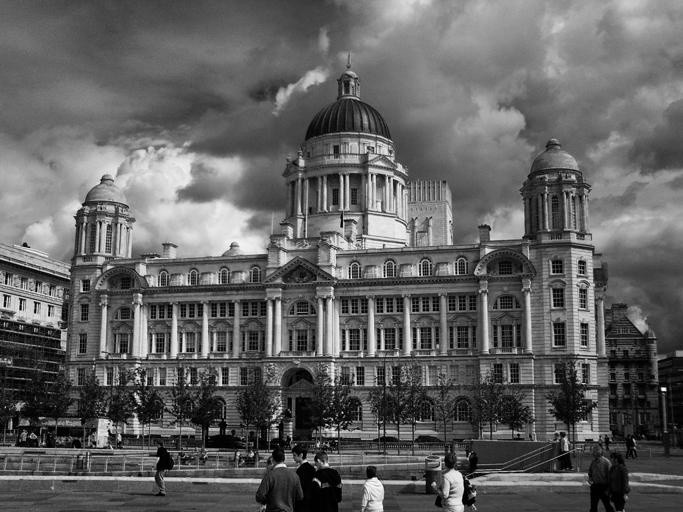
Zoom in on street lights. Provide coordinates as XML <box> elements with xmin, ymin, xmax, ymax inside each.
<box><xmin>96</xmin><ymin>349</ymin><xmax>115</xmax><ymax>419</ymax></box>
<box><xmin>657</xmin><ymin>382</ymin><xmax>667</xmax><ymax>455</ymax></box>
<box><xmin>382</xmin><ymin>347</ymin><xmax>400</xmax><ymax>455</ymax></box>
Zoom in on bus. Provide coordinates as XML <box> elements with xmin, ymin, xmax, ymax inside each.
<box><xmin>42</xmin><ymin>419</ymin><xmax>111</xmax><ymax>450</ymax></box>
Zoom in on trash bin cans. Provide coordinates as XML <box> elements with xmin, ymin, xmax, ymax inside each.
<box><xmin>425</xmin><ymin>455</ymin><xmax>442</xmax><ymax>495</ymax></box>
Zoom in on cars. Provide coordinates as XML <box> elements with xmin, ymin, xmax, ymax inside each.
<box><xmin>124</xmin><ymin>433</ymin><xmax>339</xmax><ymax>453</ymax></box>
<box><xmin>414</xmin><ymin>436</ymin><xmax>443</xmax><ymax>447</ymax></box>
<box><xmin>372</xmin><ymin>437</ymin><xmax>400</xmax><ymax>443</ymax></box>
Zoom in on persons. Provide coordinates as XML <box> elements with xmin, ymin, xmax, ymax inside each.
<box><xmin>255</xmin><ymin>449</ymin><xmax>303</xmax><ymax>512</ymax></box>
<box><xmin>218</xmin><ymin>419</ymin><xmax>227</xmax><ymax>435</ymax></box>
<box><xmin>177</xmin><ymin>447</ymin><xmax>209</xmax><ymax>465</ymax></box>
<box><xmin>469</xmin><ymin>450</ymin><xmax>476</xmax><ymax>475</ymax></box>
<box><xmin>552</xmin><ymin>432</ymin><xmax>572</xmax><ymax>470</ymax></box>
<box><xmin>598</xmin><ymin>435</ymin><xmax>609</xmax><ymax>450</ymax></box>
<box><xmin>587</xmin><ymin>444</ymin><xmax>629</xmax><ymax>511</ymax></box>
<box><xmin>313</xmin><ymin>439</ymin><xmax>338</xmax><ymax>453</ymax></box>
<box><xmin>309</xmin><ymin>451</ymin><xmax>342</xmax><ymax>511</ymax></box>
<box><xmin>279</xmin><ymin>436</ymin><xmax>292</xmax><ymax>450</ymax></box>
<box><xmin>431</xmin><ymin>453</ymin><xmax>465</xmax><ymax>511</ymax></box>
<box><xmin>71</xmin><ymin>436</ymin><xmax>81</xmax><ymax>448</ymax></box>
<box><xmin>148</xmin><ymin>439</ymin><xmax>170</xmax><ymax>497</ymax></box>
<box><xmin>671</xmin><ymin>423</ymin><xmax>679</xmax><ymax>449</ymax></box>
<box><xmin>116</xmin><ymin>433</ymin><xmax>123</xmax><ymax>448</ymax></box>
<box><xmin>361</xmin><ymin>466</ymin><xmax>384</xmax><ymax>511</ymax></box>
<box><xmin>18</xmin><ymin>429</ymin><xmax>57</xmax><ymax>448</ymax></box>
<box><xmin>626</xmin><ymin>435</ymin><xmax>638</xmax><ymax>459</ymax></box>
<box><xmin>291</xmin><ymin>445</ymin><xmax>316</xmax><ymax>511</ymax></box>
<box><xmin>239</xmin><ymin>449</ymin><xmax>254</xmax><ymax>467</ymax></box>
